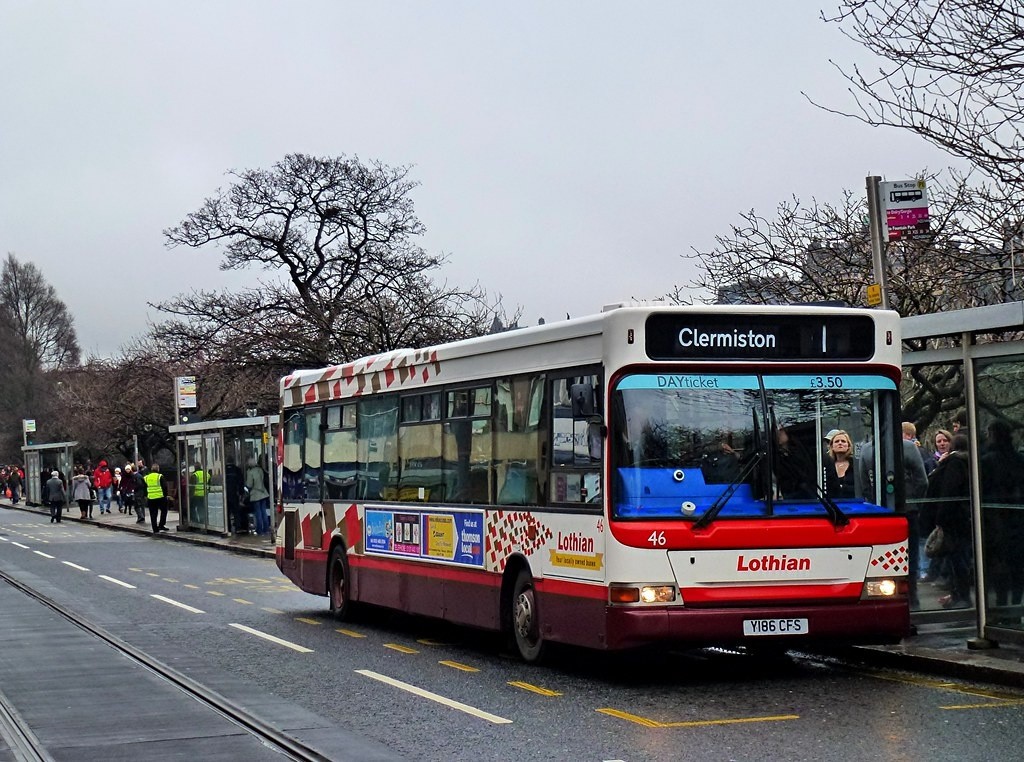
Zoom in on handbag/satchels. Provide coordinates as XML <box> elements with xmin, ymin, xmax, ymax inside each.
<box><xmin>924</xmin><ymin>525</ymin><xmax>952</xmax><ymax>558</ymax></box>
<box><xmin>5</xmin><ymin>487</ymin><xmax>13</xmax><ymax>498</ymax></box>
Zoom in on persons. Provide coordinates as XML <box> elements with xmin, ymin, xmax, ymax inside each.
<box><xmin>622</xmin><ymin>406</ymin><xmax>659</xmax><ymax>466</ymax></box>
<box><xmin>226</xmin><ymin>452</ymin><xmax>278</xmax><ymax>535</ymax></box>
<box><xmin>70</xmin><ymin>461</ymin><xmax>95</xmax><ymax>519</ymax></box>
<box><xmin>703</xmin><ymin>424</ymin><xmax>733</xmax><ymax>455</ymax></box>
<box><xmin>0</xmin><ymin>464</ymin><xmax>25</xmax><ymax>505</ymax></box>
<box><xmin>144</xmin><ymin>463</ymin><xmax>170</xmax><ymax>533</ymax></box>
<box><xmin>41</xmin><ymin>466</ymin><xmax>66</xmax><ymax>523</ymax></box>
<box><xmin>777</xmin><ymin>418</ymin><xmax>817</xmax><ymax>498</ymax></box>
<box><xmin>825</xmin><ymin>432</ymin><xmax>858</xmax><ymax>498</ymax></box>
<box><xmin>113</xmin><ymin>460</ymin><xmax>148</xmax><ymax>523</ymax></box>
<box><xmin>858</xmin><ymin>438</ymin><xmax>928</xmax><ymax>609</ymax></box>
<box><xmin>182</xmin><ymin>462</ymin><xmax>212</xmax><ymax>524</ymax></box>
<box><xmin>901</xmin><ymin>408</ymin><xmax>1024</xmax><ymax>610</ymax></box>
<box><xmin>93</xmin><ymin>461</ymin><xmax>112</xmax><ymax>515</ymax></box>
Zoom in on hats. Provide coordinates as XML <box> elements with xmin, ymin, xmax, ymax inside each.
<box><xmin>125</xmin><ymin>465</ymin><xmax>131</xmax><ymax>470</ymax></box>
<box><xmin>51</xmin><ymin>471</ymin><xmax>59</xmax><ymax>479</ymax></box>
<box><xmin>824</xmin><ymin>430</ymin><xmax>840</xmax><ymax>441</ymax></box>
<box><xmin>115</xmin><ymin>468</ymin><xmax>121</xmax><ymax>473</ymax></box>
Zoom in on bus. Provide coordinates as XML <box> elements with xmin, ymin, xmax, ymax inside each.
<box><xmin>274</xmin><ymin>298</ymin><xmax>913</xmax><ymax>673</ymax></box>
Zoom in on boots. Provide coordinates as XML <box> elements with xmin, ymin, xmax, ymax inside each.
<box><xmin>81</xmin><ymin>511</ymin><xmax>86</xmax><ymax>518</ymax></box>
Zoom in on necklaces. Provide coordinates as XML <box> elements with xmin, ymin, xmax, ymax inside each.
<box><xmin>839</xmin><ymin>463</ymin><xmax>843</xmax><ymax>468</ymax></box>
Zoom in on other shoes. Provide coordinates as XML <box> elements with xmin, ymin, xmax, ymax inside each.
<box><xmin>256</xmin><ymin>528</ymin><xmax>262</xmax><ymax>534</ymax></box>
<box><xmin>154</xmin><ymin>529</ymin><xmax>159</xmax><ymax>533</ymax></box>
<box><xmin>57</xmin><ymin>519</ymin><xmax>61</xmax><ymax>523</ymax></box>
<box><xmin>917</xmin><ymin>572</ymin><xmax>937</xmax><ymax>585</ymax></box>
<box><xmin>930</xmin><ymin>576</ymin><xmax>951</xmax><ymax>591</ymax></box>
<box><xmin>263</xmin><ymin>527</ymin><xmax>272</xmax><ymax>532</ymax></box>
<box><xmin>100</xmin><ymin>506</ymin><xmax>133</xmax><ymax>515</ymax></box>
<box><xmin>987</xmin><ymin>615</ymin><xmax>1021</xmax><ymax>626</ymax></box>
<box><xmin>51</xmin><ymin>515</ymin><xmax>55</xmax><ymax>523</ymax></box>
<box><xmin>90</xmin><ymin>515</ymin><xmax>92</xmax><ymax>519</ymax></box>
<box><xmin>236</xmin><ymin>529</ymin><xmax>248</xmax><ymax>535</ymax></box>
<box><xmin>943</xmin><ymin>598</ymin><xmax>970</xmax><ymax>608</ymax></box>
<box><xmin>136</xmin><ymin>513</ymin><xmax>144</xmax><ymax>523</ymax></box>
<box><xmin>938</xmin><ymin>594</ymin><xmax>953</xmax><ymax>605</ymax></box>
<box><xmin>158</xmin><ymin>524</ymin><xmax>169</xmax><ymax>530</ymax></box>
